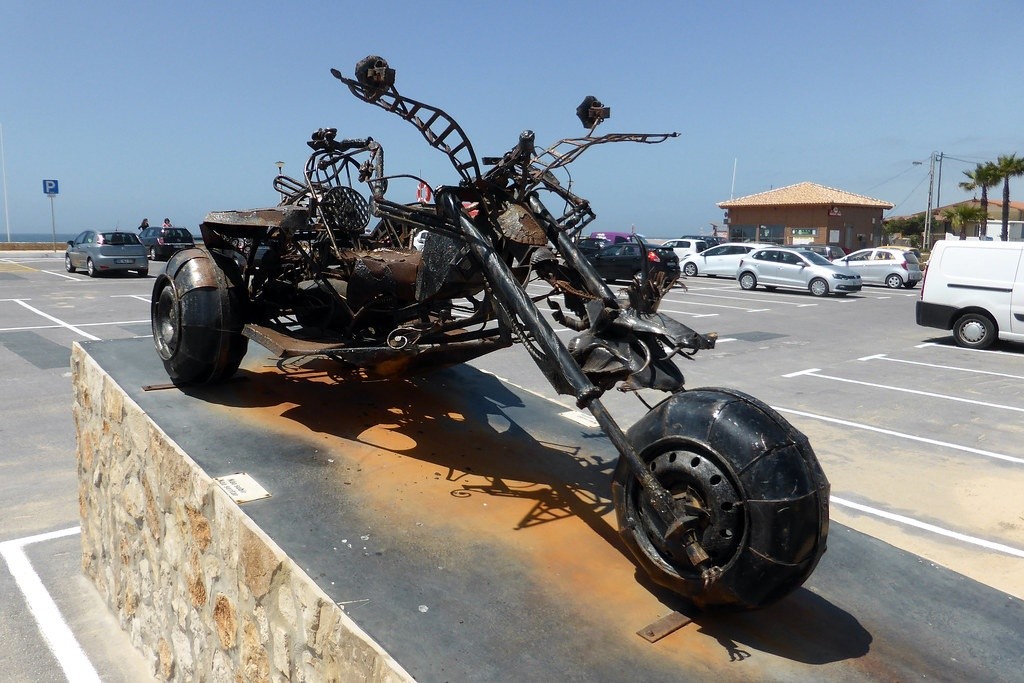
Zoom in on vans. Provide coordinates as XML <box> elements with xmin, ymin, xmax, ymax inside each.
<box><xmin>590</xmin><ymin>232</ymin><xmax>649</xmax><ymax>251</ymax></box>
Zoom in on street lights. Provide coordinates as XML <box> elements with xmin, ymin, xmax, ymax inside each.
<box><xmin>912</xmin><ymin>162</ymin><xmax>934</xmax><ymax>249</ymax></box>
<box><xmin>275</xmin><ymin>161</ymin><xmax>285</xmax><ymax>202</ymax></box>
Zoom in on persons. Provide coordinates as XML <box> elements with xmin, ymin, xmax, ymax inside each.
<box><xmin>138</xmin><ymin>218</ymin><xmax>150</xmax><ymax>232</ymax></box>
<box><xmin>162</xmin><ymin>217</ymin><xmax>172</xmax><ymax>227</ymax></box>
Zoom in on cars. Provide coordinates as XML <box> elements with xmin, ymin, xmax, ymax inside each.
<box><xmin>736</xmin><ymin>247</ymin><xmax>863</xmax><ymax>297</ymax></box>
<box><xmin>831</xmin><ymin>248</ymin><xmax>923</xmax><ymax>289</ymax></box>
<box><xmin>661</xmin><ymin>239</ymin><xmax>708</xmax><ymax>262</ymax></box>
<box><xmin>574</xmin><ymin>238</ymin><xmax>611</xmax><ymax>255</ymax></box>
<box><xmin>584</xmin><ymin>242</ymin><xmax>681</xmax><ymax>282</ymax></box>
<box><xmin>784</xmin><ymin>245</ymin><xmax>846</xmax><ymax>262</ymax></box>
<box><xmin>65</xmin><ymin>230</ymin><xmax>149</xmax><ymax>277</ymax></box>
<box><xmin>682</xmin><ymin>235</ymin><xmax>730</xmax><ymax>254</ymax></box>
<box><xmin>864</xmin><ymin>245</ymin><xmax>925</xmax><ymax>271</ymax></box>
<box><xmin>138</xmin><ymin>227</ymin><xmax>195</xmax><ymax>261</ymax></box>
<box><xmin>679</xmin><ymin>241</ymin><xmax>778</xmax><ymax>277</ymax></box>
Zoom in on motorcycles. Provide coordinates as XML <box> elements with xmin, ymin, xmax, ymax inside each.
<box><xmin>151</xmin><ymin>55</ymin><xmax>831</xmax><ymax>613</ymax></box>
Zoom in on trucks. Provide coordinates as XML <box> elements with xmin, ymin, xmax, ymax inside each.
<box><xmin>916</xmin><ymin>240</ymin><xmax>1024</xmax><ymax>350</ymax></box>
